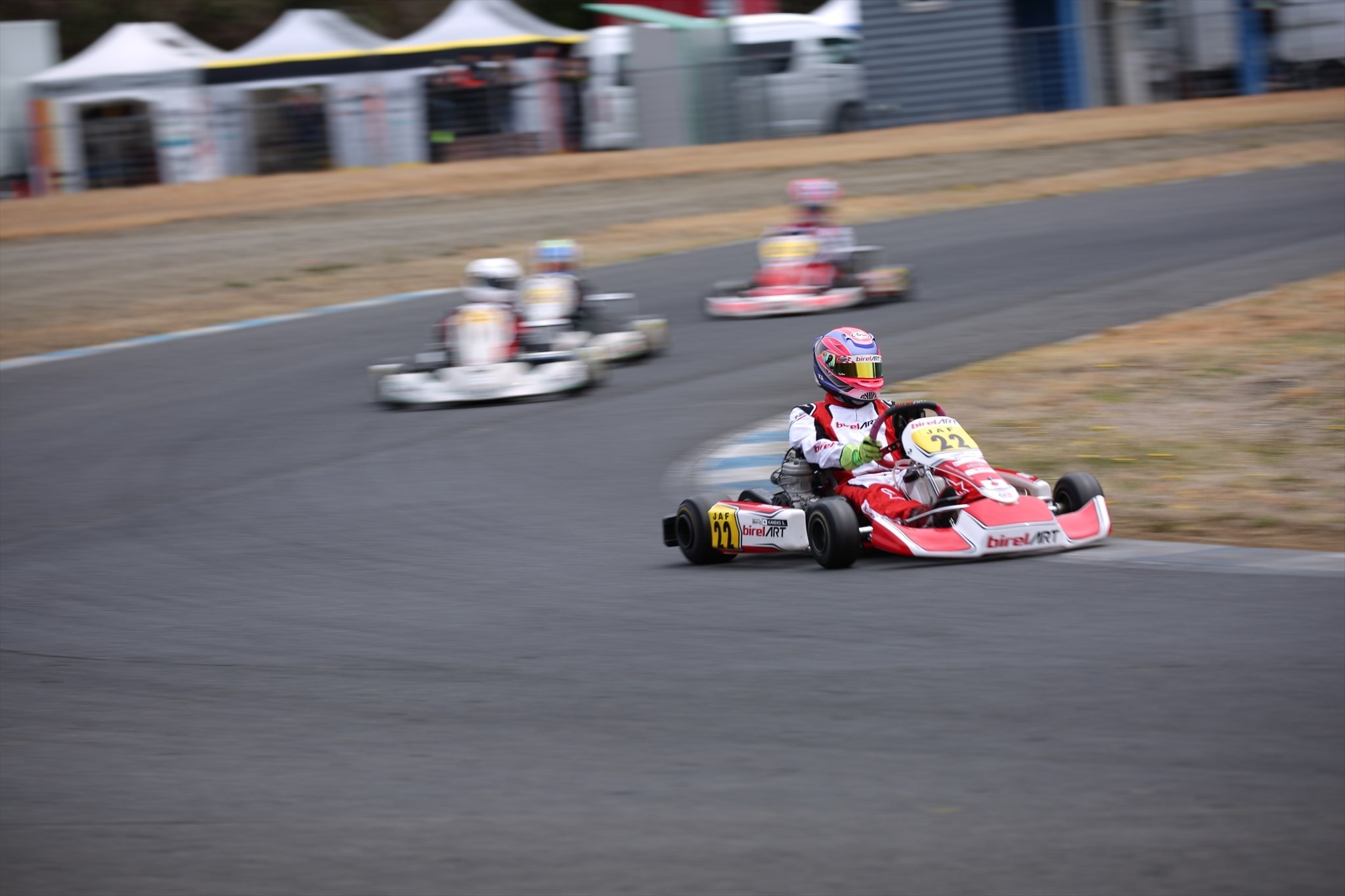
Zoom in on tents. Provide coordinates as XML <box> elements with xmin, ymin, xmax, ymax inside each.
<box><xmin>20</xmin><ymin>0</ymin><xmax>593</xmax><ymax>194</ymax></box>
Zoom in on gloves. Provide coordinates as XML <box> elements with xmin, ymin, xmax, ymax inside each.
<box><xmin>839</xmin><ymin>436</ymin><xmax>883</xmax><ymax>471</ymax></box>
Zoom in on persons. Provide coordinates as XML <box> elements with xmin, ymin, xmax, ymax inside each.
<box><xmin>788</xmin><ymin>327</ymin><xmax>961</xmax><ymax>529</ymax></box>
<box><xmin>438</xmin><ymin>255</ymin><xmax>525</xmax><ymax>366</ymax></box>
<box><xmin>788</xmin><ymin>178</ymin><xmax>855</xmax><ymax>287</ymax></box>
<box><xmin>536</xmin><ymin>241</ymin><xmax>597</xmax><ymax>331</ymax></box>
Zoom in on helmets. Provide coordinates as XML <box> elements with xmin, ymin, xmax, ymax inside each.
<box><xmin>462</xmin><ymin>258</ymin><xmax>521</xmax><ymax>303</ymax></box>
<box><xmin>813</xmin><ymin>327</ymin><xmax>884</xmax><ymax>401</ymax></box>
<box><xmin>531</xmin><ymin>239</ymin><xmax>580</xmax><ymax>276</ymax></box>
<box><xmin>787</xmin><ymin>178</ymin><xmax>843</xmax><ymax>220</ymax></box>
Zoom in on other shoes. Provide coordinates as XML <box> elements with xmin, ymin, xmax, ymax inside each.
<box><xmin>916</xmin><ymin>486</ymin><xmax>957</xmax><ymax>529</ymax></box>
